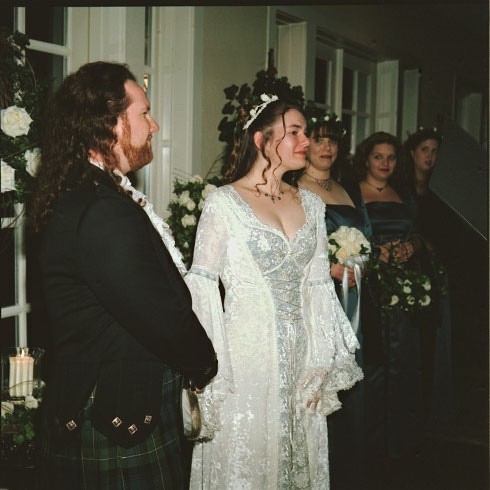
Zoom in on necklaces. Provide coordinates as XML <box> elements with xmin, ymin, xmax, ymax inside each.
<box><xmin>247</xmin><ymin>174</ymin><xmax>285</xmax><ymax>201</ymax></box>
<box><xmin>365</xmin><ymin>179</ymin><xmax>388</xmax><ymax>192</ymax></box>
<box><xmin>305</xmin><ymin>172</ymin><xmax>333</xmax><ymax>192</ymax></box>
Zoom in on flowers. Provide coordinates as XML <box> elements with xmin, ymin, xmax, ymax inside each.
<box><xmin>327</xmin><ymin>225</ymin><xmax>371</xmax><ymax>290</ymax></box>
<box><xmin>0</xmin><ymin>378</ymin><xmax>47</xmax><ymax>444</ymax></box>
<box><xmin>0</xmin><ymin>31</ymin><xmax>54</xmax><ymax>228</ymax></box>
<box><xmin>162</xmin><ymin>169</ymin><xmax>225</xmax><ymax>270</ymax></box>
<box><xmin>376</xmin><ymin>242</ymin><xmax>432</xmax><ymax>314</ymax></box>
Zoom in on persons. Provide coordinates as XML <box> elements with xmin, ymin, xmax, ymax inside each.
<box><xmin>389</xmin><ymin>128</ymin><xmax>456</xmax><ymax>422</ymax></box>
<box><xmin>287</xmin><ymin>111</ymin><xmax>364</xmax><ymax>467</ymax></box>
<box><xmin>22</xmin><ymin>60</ymin><xmax>219</xmax><ymax>490</ymax></box>
<box><xmin>340</xmin><ymin>131</ymin><xmax>424</xmax><ymax>459</ymax></box>
<box><xmin>181</xmin><ymin>91</ymin><xmax>363</xmax><ymax>490</ymax></box>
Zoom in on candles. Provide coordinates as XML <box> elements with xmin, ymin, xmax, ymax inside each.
<box><xmin>10</xmin><ymin>350</ymin><xmax>34</xmax><ymax>399</ymax></box>
